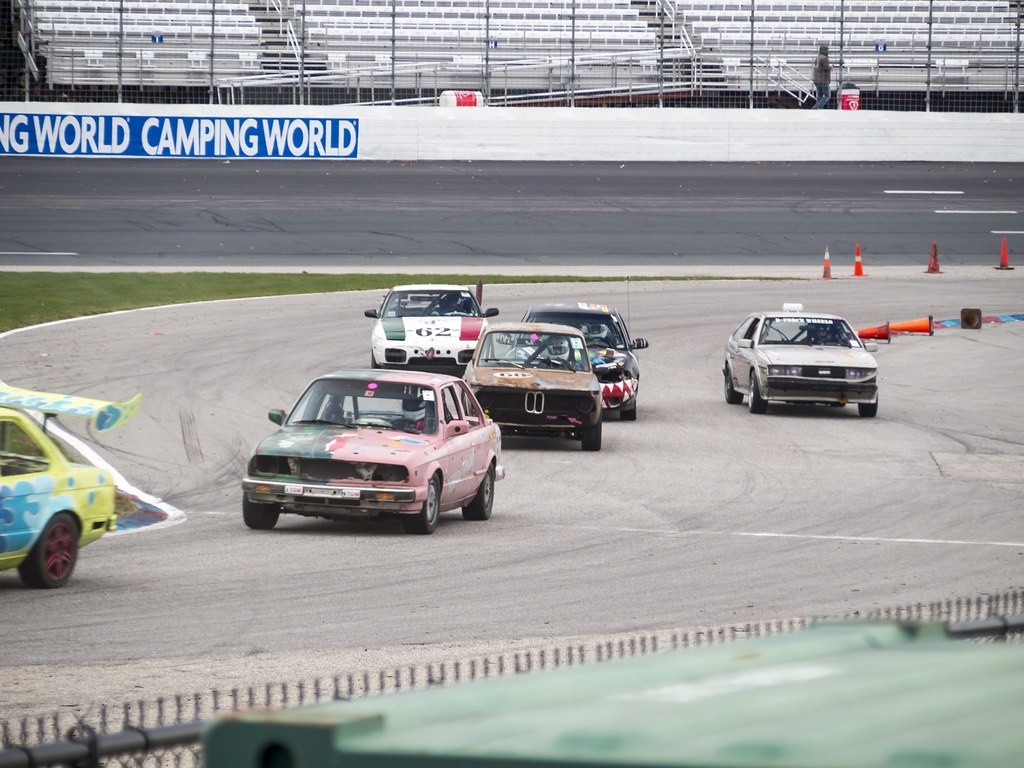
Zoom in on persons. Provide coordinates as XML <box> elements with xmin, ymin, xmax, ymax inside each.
<box><xmin>800</xmin><ymin>323</ymin><xmax>835</xmax><ymax>344</ymax></box>
<box><xmin>400</xmin><ymin>397</ymin><xmax>427</xmax><ymax>431</ymax></box>
<box><xmin>543</xmin><ymin>337</ymin><xmax>571</xmax><ymax>369</ymax></box>
<box><xmin>810</xmin><ymin>45</ymin><xmax>831</xmax><ymax>106</ymax></box>
<box><xmin>583</xmin><ymin>323</ymin><xmax>609</xmax><ymax>348</ymax></box>
<box><xmin>431</xmin><ymin>296</ymin><xmax>466</xmax><ymax>316</ymax></box>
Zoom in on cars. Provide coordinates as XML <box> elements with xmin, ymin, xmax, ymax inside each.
<box><xmin>461</xmin><ymin>321</ymin><xmax>606</xmax><ymax>451</ymax></box>
<box><xmin>722</xmin><ymin>302</ymin><xmax>880</xmax><ymax>418</ymax></box>
<box><xmin>362</xmin><ymin>283</ymin><xmax>499</xmax><ymax>377</ymax></box>
<box><xmin>0</xmin><ymin>380</ymin><xmax>144</xmax><ymax>589</ymax></box>
<box><xmin>520</xmin><ymin>300</ymin><xmax>649</xmax><ymax>422</ymax></box>
<box><xmin>241</xmin><ymin>366</ymin><xmax>506</xmax><ymax>536</ymax></box>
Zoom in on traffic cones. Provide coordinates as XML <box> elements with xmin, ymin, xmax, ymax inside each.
<box><xmin>848</xmin><ymin>243</ymin><xmax>868</xmax><ymax>276</ymax></box>
<box><xmin>819</xmin><ymin>245</ymin><xmax>838</xmax><ymax>280</ymax></box>
<box><xmin>923</xmin><ymin>239</ymin><xmax>945</xmax><ymax>273</ymax></box>
<box><xmin>993</xmin><ymin>236</ymin><xmax>1015</xmax><ymax>270</ymax></box>
<box><xmin>960</xmin><ymin>307</ymin><xmax>983</xmax><ymax>330</ymax></box>
<box><xmin>888</xmin><ymin>315</ymin><xmax>934</xmax><ymax>337</ymax></box>
<box><xmin>854</xmin><ymin>320</ymin><xmax>891</xmax><ymax>344</ymax></box>
